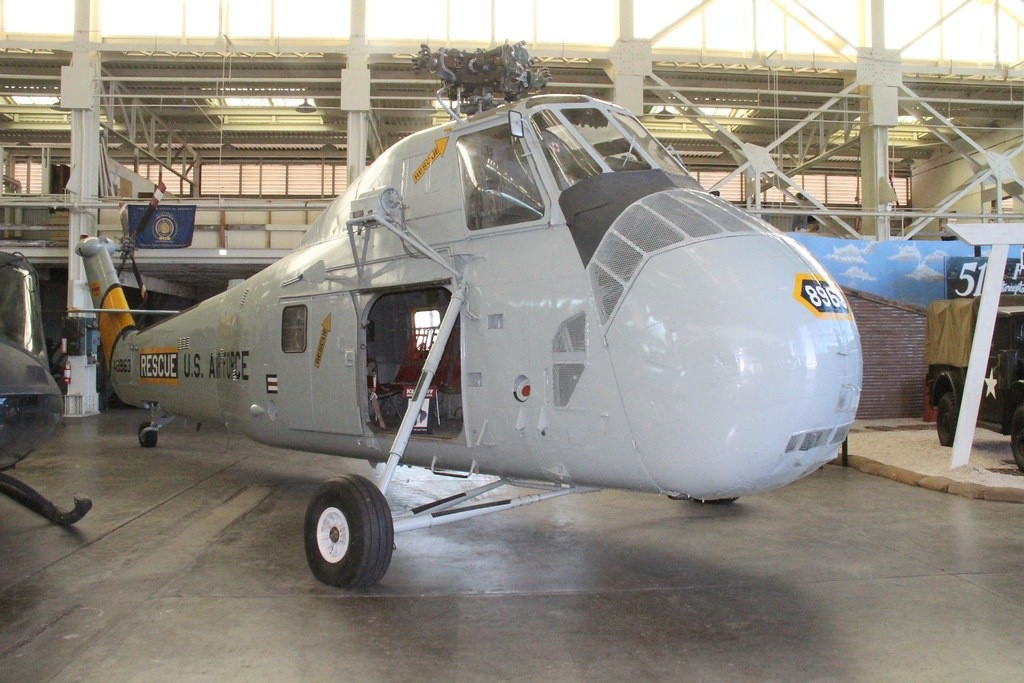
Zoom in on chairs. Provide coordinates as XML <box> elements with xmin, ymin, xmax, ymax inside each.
<box><xmin>367</xmin><ymin>372</ymin><xmax>403</xmax><ymax>422</ymax></box>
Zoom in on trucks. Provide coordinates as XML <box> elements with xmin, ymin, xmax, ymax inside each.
<box><xmin>925</xmin><ymin>291</ymin><xmax>1024</xmax><ymax>475</ymax></box>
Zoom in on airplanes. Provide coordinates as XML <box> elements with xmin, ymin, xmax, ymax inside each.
<box><xmin>74</xmin><ymin>44</ymin><xmax>871</xmax><ymax>593</ymax></box>
<box><xmin>1</xmin><ymin>251</ymin><xmax>94</xmax><ymax>526</ymax></box>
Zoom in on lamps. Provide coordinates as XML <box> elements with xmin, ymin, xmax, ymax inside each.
<box><xmin>654</xmin><ymin>105</ymin><xmax>675</xmax><ymax>120</ymax></box>
<box><xmin>295</xmin><ymin>99</ymin><xmax>316</xmax><ymax>113</ymax></box>
